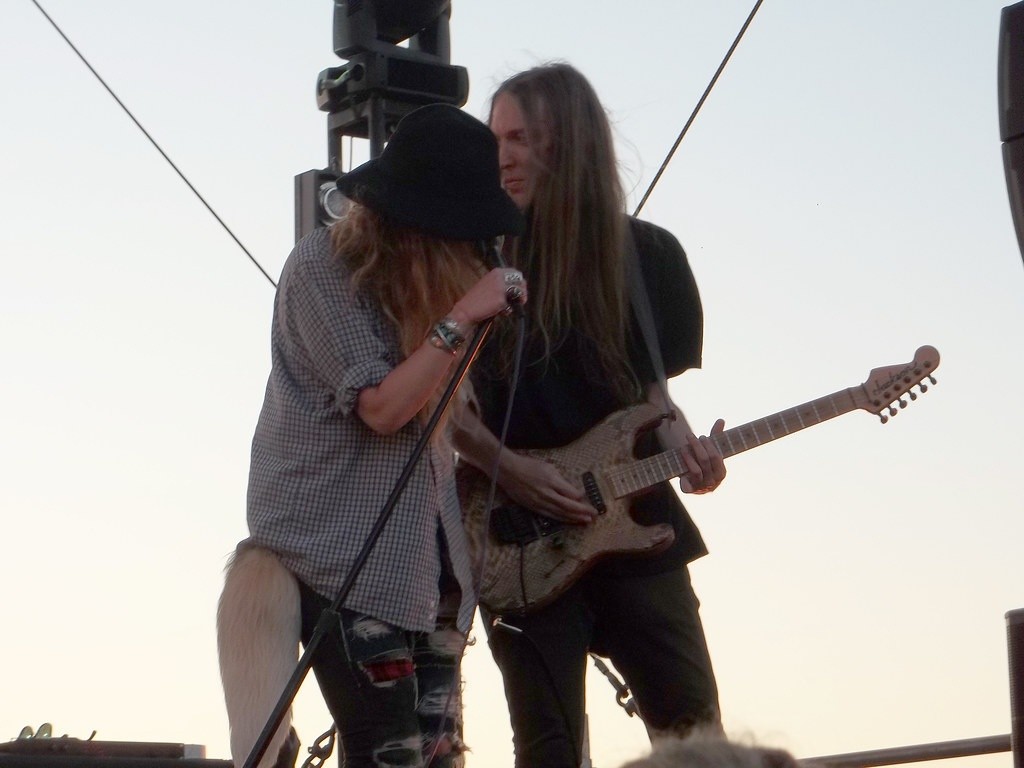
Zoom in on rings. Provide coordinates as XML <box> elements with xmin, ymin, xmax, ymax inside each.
<box><xmin>505</xmin><ymin>285</ymin><xmax>522</xmax><ymax>301</ymax></box>
<box><xmin>504</xmin><ymin>272</ymin><xmax>522</xmax><ymax>285</ymax></box>
<box><xmin>505</xmin><ymin>305</ymin><xmax>511</xmax><ymax>312</ymax></box>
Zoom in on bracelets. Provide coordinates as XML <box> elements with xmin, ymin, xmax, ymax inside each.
<box><xmin>430</xmin><ymin>318</ymin><xmax>468</xmax><ymax>357</ymax></box>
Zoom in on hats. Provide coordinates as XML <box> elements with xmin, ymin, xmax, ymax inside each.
<box><xmin>336</xmin><ymin>103</ymin><xmax>525</xmax><ymax>243</ymax></box>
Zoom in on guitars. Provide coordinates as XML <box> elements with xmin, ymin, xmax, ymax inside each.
<box><xmin>442</xmin><ymin>344</ymin><xmax>942</xmax><ymax>616</ymax></box>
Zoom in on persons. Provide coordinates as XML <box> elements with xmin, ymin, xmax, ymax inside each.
<box><xmin>444</xmin><ymin>65</ymin><xmax>726</xmax><ymax>768</ymax></box>
<box><xmin>218</xmin><ymin>104</ymin><xmax>528</xmax><ymax>768</ymax></box>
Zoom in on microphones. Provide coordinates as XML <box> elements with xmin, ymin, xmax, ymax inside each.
<box><xmin>471</xmin><ymin>235</ymin><xmax>527</xmax><ymax>318</ymax></box>
